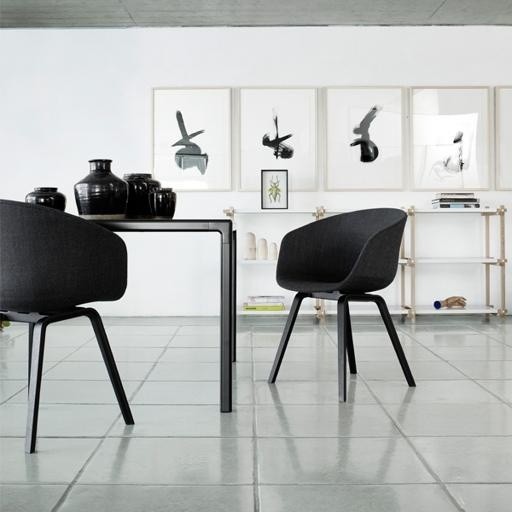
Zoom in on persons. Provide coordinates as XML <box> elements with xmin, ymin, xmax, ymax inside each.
<box><xmin>433</xmin><ymin>295</ymin><xmax>467</xmax><ymax>310</ymax></box>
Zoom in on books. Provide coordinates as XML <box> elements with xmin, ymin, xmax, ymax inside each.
<box><xmin>431</xmin><ymin>203</ymin><xmax>480</xmax><ymax>208</ymax></box>
<box><xmin>431</xmin><ymin>197</ymin><xmax>479</xmax><ymax>204</ymax></box>
<box><xmin>248</xmin><ymin>301</ymin><xmax>281</xmax><ymax>306</ymax></box>
<box><xmin>247</xmin><ymin>295</ymin><xmax>285</xmax><ymax>302</ymax></box>
<box><xmin>435</xmin><ymin>192</ymin><xmax>474</xmax><ymax>198</ymax></box>
<box><xmin>243</xmin><ymin>302</ymin><xmax>285</xmax><ymax>311</ymax></box>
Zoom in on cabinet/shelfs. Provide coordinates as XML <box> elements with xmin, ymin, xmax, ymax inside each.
<box><xmin>226</xmin><ymin>208</ymin><xmax>506</xmax><ymax>331</ymax></box>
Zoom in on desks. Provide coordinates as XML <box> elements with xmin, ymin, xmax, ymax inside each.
<box><xmin>78</xmin><ymin>218</ymin><xmax>239</xmax><ymax>415</ymax></box>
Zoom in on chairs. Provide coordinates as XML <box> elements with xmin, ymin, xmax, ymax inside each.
<box><xmin>0</xmin><ymin>199</ymin><xmax>133</xmax><ymax>455</ymax></box>
<box><xmin>266</xmin><ymin>207</ymin><xmax>417</xmax><ymax>403</ymax></box>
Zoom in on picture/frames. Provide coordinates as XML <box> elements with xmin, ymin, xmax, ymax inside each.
<box><xmin>494</xmin><ymin>88</ymin><xmax>511</xmax><ymax>192</ymax></box>
<box><xmin>239</xmin><ymin>88</ymin><xmax>318</xmax><ymax>191</ymax></box>
<box><xmin>152</xmin><ymin>87</ymin><xmax>233</xmax><ymax>191</ymax></box>
<box><xmin>260</xmin><ymin>169</ymin><xmax>289</xmax><ymax>208</ymax></box>
<box><xmin>325</xmin><ymin>88</ymin><xmax>403</xmax><ymax>191</ymax></box>
<box><xmin>408</xmin><ymin>87</ymin><xmax>493</xmax><ymax>191</ymax></box>
